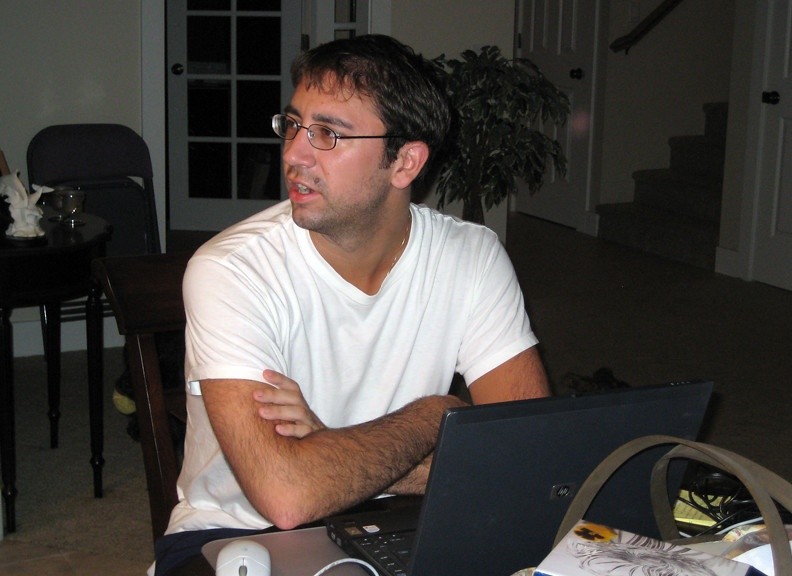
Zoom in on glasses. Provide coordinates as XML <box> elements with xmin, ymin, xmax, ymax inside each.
<box><xmin>271</xmin><ymin>112</ymin><xmax>410</xmax><ymax>151</ymax></box>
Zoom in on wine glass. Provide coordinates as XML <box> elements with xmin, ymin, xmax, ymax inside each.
<box><xmin>48</xmin><ymin>185</ymin><xmax>75</xmax><ymax>222</ymax></box>
<box><xmin>58</xmin><ymin>190</ymin><xmax>88</xmax><ymax>226</ymax></box>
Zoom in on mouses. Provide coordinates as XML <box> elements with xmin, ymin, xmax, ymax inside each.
<box><xmin>216</xmin><ymin>539</ymin><xmax>271</xmax><ymax>576</ymax></box>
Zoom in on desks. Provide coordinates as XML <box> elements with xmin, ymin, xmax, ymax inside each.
<box><xmin>0</xmin><ymin>205</ymin><xmax>113</xmax><ymax>528</ymax></box>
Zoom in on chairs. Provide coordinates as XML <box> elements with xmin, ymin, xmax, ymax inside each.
<box><xmin>93</xmin><ymin>254</ymin><xmax>190</xmax><ymax>541</ymax></box>
<box><xmin>27</xmin><ymin>122</ymin><xmax>161</xmax><ymax>447</ymax></box>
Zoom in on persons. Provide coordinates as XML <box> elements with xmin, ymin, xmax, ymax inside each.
<box><xmin>155</xmin><ymin>35</ymin><xmax>553</xmax><ymax>575</ymax></box>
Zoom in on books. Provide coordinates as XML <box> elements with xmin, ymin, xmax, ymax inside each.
<box><xmin>533</xmin><ymin>519</ymin><xmax>751</xmax><ymax>575</ymax></box>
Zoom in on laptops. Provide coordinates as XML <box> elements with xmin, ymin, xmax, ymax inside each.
<box><xmin>326</xmin><ymin>378</ymin><xmax>715</xmax><ymax>576</ymax></box>
